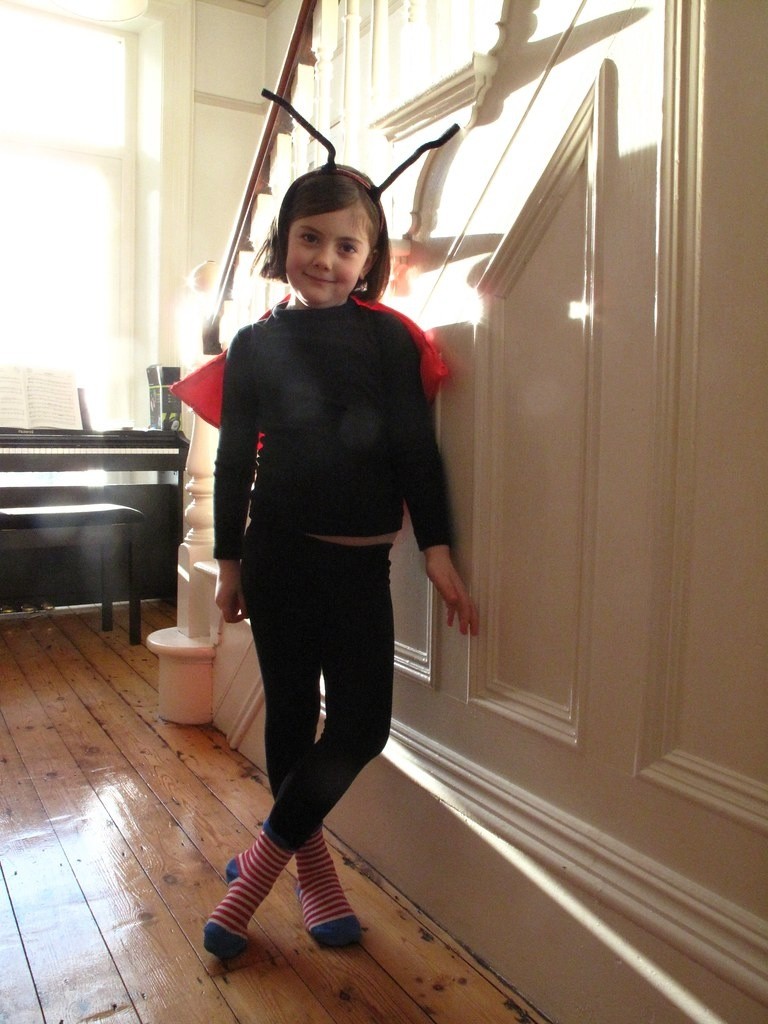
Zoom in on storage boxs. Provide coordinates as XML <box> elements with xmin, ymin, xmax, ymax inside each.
<box><xmin>147</xmin><ymin>366</ymin><xmax>182</xmax><ymax>431</ymax></box>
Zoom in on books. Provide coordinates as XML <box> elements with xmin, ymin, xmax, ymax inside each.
<box><xmin>0</xmin><ymin>366</ymin><xmax>84</xmax><ymax>431</ymax></box>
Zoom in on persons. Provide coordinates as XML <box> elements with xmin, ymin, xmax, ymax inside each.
<box><xmin>203</xmin><ymin>163</ymin><xmax>480</xmax><ymax>960</ymax></box>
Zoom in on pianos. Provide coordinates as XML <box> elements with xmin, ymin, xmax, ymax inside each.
<box><xmin>0</xmin><ymin>428</ymin><xmax>190</xmax><ymax>646</ymax></box>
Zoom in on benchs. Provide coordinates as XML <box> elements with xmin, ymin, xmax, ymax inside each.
<box><xmin>0</xmin><ymin>503</ymin><xmax>143</xmax><ymax>644</ymax></box>
